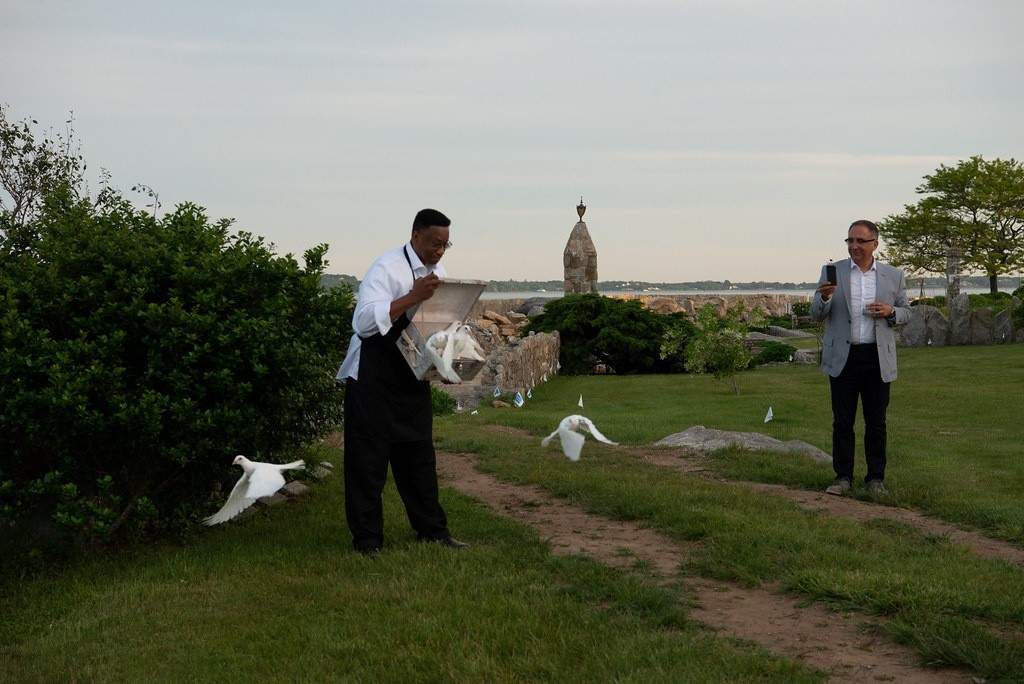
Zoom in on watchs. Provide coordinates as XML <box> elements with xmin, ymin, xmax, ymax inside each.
<box><xmin>887</xmin><ymin>306</ymin><xmax>895</xmax><ymax>318</ymax></box>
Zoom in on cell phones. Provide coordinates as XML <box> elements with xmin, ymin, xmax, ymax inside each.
<box><xmin>826</xmin><ymin>265</ymin><xmax>837</xmax><ymax>287</ymax></box>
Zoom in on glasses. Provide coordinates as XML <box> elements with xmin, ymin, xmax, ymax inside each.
<box><xmin>845</xmin><ymin>238</ymin><xmax>876</xmax><ymax>243</ymax></box>
<box><xmin>418</xmin><ymin>232</ymin><xmax>453</xmax><ymax>249</ymax></box>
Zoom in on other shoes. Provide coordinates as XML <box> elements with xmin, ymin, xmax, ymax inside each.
<box><xmin>826</xmin><ymin>479</ymin><xmax>850</xmax><ymax>495</ymax></box>
<box><xmin>360</xmin><ymin>547</ymin><xmax>379</xmax><ymax>559</ymax></box>
<box><xmin>865</xmin><ymin>477</ymin><xmax>889</xmax><ymax>502</ymax></box>
<box><xmin>415</xmin><ymin>532</ymin><xmax>468</xmax><ymax>549</ymax></box>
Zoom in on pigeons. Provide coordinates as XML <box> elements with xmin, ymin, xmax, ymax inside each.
<box><xmin>541</xmin><ymin>414</ymin><xmax>620</xmax><ymax>462</ymax></box>
<box><xmin>425</xmin><ymin>321</ymin><xmax>487</xmax><ymax>384</ymax></box>
<box><xmin>202</xmin><ymin>455</ymin><xmax>306</xmax><ymax>526</ymax></box>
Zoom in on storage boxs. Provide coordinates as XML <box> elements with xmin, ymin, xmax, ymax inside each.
<box><xmin>395</xmin><ymin>279</ymin><xmax>488</xmax><ymax>382</ymax></box>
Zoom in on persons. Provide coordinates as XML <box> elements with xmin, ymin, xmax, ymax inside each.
<box><xmin>808</xmin><ymin>219</ymin><xmax>914</xmax><ymax>498</ymax></box>
<box><xmin>335</xmin><ymin>207</ymin><xmax>469</xmax><ymax>560</ymax></box>
